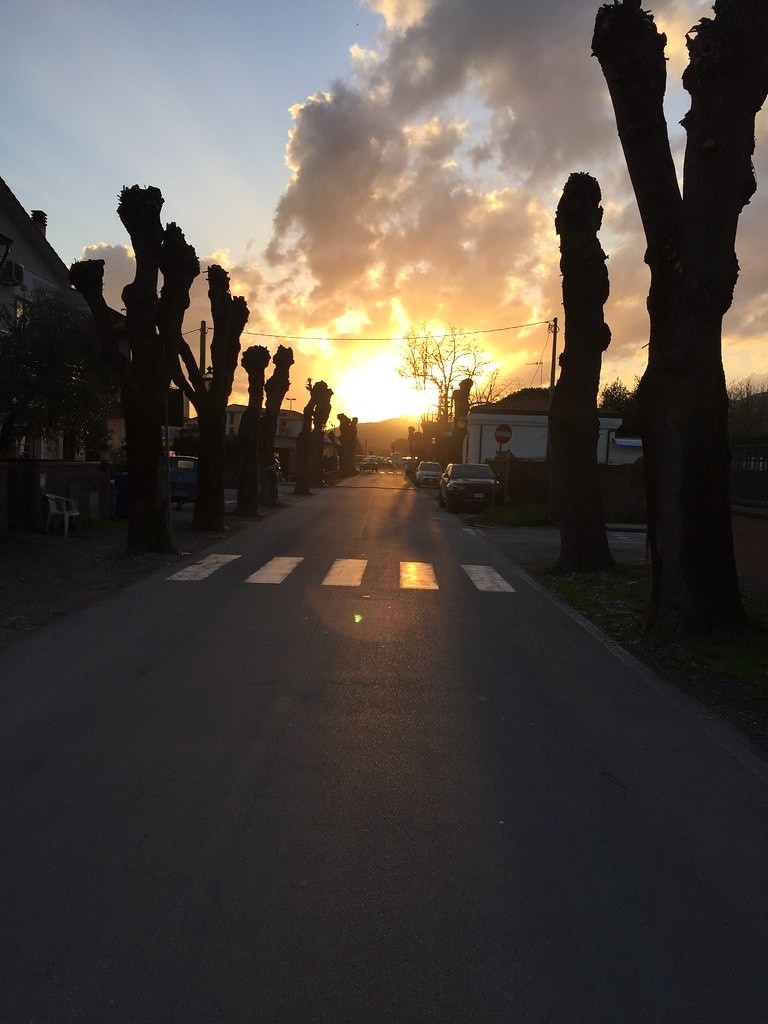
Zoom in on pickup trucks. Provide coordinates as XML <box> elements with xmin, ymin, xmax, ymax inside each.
<box><xmin>359</xmin><ymin>458</ymin><xmax>378</xmax><ymax>472</ymax></box>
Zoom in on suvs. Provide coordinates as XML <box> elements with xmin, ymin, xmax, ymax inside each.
<box><xmin>415</xmin><ymin>461</ymin><xmax>443</xmax><ymax>488</ymax></box>
<box><xmin>439</xmin><ymin>462</ymin><xmax>504</xmax><ymax>512</ymax></box>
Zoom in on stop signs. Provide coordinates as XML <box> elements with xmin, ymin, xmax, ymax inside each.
<box><xmin>494</xmin><ymin>424</ymin><xmax>512</xmax><ymax>443</ymax></box>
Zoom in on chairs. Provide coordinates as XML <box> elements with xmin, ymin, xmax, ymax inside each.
<box><xmin>42</xmin><ymin>492</ymin><xmax>80</xmax><ymax>539</ymax></box>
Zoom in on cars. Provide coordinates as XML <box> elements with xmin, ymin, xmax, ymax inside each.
<box><xmin>406</xmin><ymin>461</ymin><xmax>419</xmax><ymax>475</ymax></box>
<box><xmin>354</xmin><ymin>454</ymin><xmax>393</xmax><ymax>464</ymax></box>
